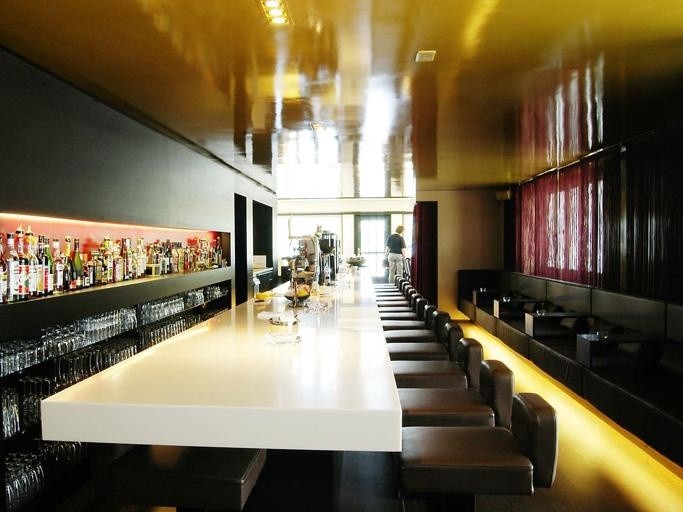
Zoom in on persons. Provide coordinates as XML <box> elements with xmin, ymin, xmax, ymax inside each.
<box><xmin>384</xmin><ymin>226</ymin><xmax>406</xmax><ymax>283</ymax></box>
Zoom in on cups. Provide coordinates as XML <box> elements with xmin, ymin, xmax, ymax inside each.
<box><xmin>75</xmin><ymin>307</ymin><xmax>138</xmax><ymax>346</ymax></box>
<box><xmin>6</xmin><ymin>439</ymin><xmax>87</xmax><ymax>505</ymax></box>
<box><xmin>141</xmin><ymin>296</ymin><xmax>185</xmax><ymax>324</ymax></box>
<box><xmin>185</xmin><ymin>287</ymin><xmax>205</xmax><ymax>308</ymax></box>
<box><xmin>0</xmin><ymin>338</ymin><xmax>44</xmax><ymax>377</ymax></box>
<box><xmin>41</xmin><ymin>323</ymin><xmax>84</xmax><ymax>358</ymax></box>
<box><xmin>206</xmin><ymin>286</ymin><xmax>221</xmax><ymax>299</ymax></box>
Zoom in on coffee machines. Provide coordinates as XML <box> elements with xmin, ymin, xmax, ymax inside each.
<box><xmin>320</xmin><ymin>229</ymin><xmax>336</xmax><ymax>286</ymax></box>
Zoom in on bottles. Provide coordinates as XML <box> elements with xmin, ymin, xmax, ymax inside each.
<box><xmin>140</xmin><ymin>312</ymin><xmax>204</xmax><ymax>351</ymax></box>
<box><xmin>0</xmin><ymin>223</ymin><xmax>222</xmax><ymax>305</ymax></box>
<box><xmin>101</xmin><ymin>341</ymin><xmax>138</xmax><ymax>367</ymax></box>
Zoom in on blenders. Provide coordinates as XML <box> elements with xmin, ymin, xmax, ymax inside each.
<box><xmin>292</xmin><ymin>239</ymin><xmax>318</xmax><ymax>285</ymax></box>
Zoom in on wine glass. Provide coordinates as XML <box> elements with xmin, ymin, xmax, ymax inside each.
<box><xmin>1</xmin><ymin>387</ymin><xmax>20</xmax><ymax>437</ymax></box>
<box><xmin>22</xmin><ymin>347</ymin><xmax>103</xmax><ymax>423</ymax></box>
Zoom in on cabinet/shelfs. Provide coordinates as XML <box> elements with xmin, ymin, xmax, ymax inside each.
<box><xmin>1</xmin><ymin>215</ymin><xmax>231</xmax><ymax>510</ymax></box>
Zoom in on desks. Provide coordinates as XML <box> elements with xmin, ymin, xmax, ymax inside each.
<box><xmin>468</xmin><ymin>282</ymin><xmax>669</xmax><ymax>371</ymax></box>
<box><xmin>39</xmin><ymin>275</ymin><xmax>403</xmax><ymax>508</ymax></box>
